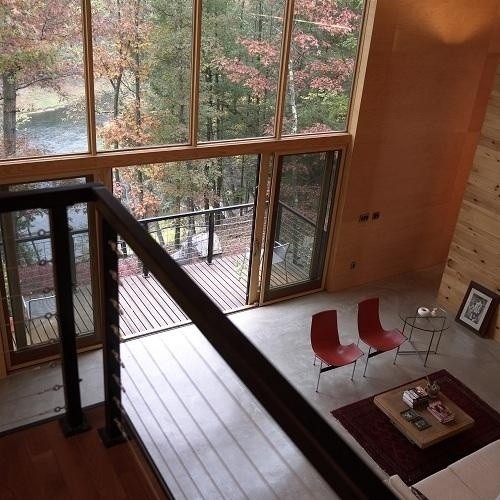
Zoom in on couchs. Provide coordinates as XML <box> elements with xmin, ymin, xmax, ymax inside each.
<box><xmin>385</xmin><ymin>439</ymin><xmax>500</xmax><ymax>499</ymax></box>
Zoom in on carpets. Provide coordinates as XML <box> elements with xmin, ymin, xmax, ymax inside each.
<box><xmin>330</xmin><ymin>368</ymin><xmax>500</xmax><ymax>488</ymax></box>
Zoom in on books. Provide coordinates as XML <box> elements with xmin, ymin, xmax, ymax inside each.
<box><xmin>399</xmin><ymin>385</ymin><xmax>456</xmax><ymax>425</ymax></box>
<box><xmin>412</xmin><ymin>416</ymin><xmax>432</xmax><ymax>431</ymax></box>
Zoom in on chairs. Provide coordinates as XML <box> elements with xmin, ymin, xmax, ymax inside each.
<box><xmin>18</xmin><ymin>291</ymin><xmax>62</xmax><ymax>347</ymax></box>
<box><xmin>309</xmin><ymin>297</ymin><xmax>409</xmax><ymax>392</ymax></box>
<box><xmin>238</xmin><ymin>233</ymin><xmax>292</xmax><ymax>287</ymax></box>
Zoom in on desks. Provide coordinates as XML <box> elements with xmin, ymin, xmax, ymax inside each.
<box><xmin>393</xmin><ymin>302</ymin><xmax>456</xmax><ymax>368</ymax></box>
<box><xmin>374</xmin><ymin>378</ymin><xmax>475</xmax><ymax>449</ymax></box>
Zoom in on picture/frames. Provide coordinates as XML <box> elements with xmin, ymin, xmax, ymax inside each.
<box><xmin>455</xmin><ymin>279</ymin><xmax>500</xmax><ymax>338</ymax></box>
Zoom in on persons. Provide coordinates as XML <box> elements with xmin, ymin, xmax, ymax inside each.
<box><xmin>469</xmin><ymin>298</ymin><xmax>484</xmax><ymax>322</ymax></box>
